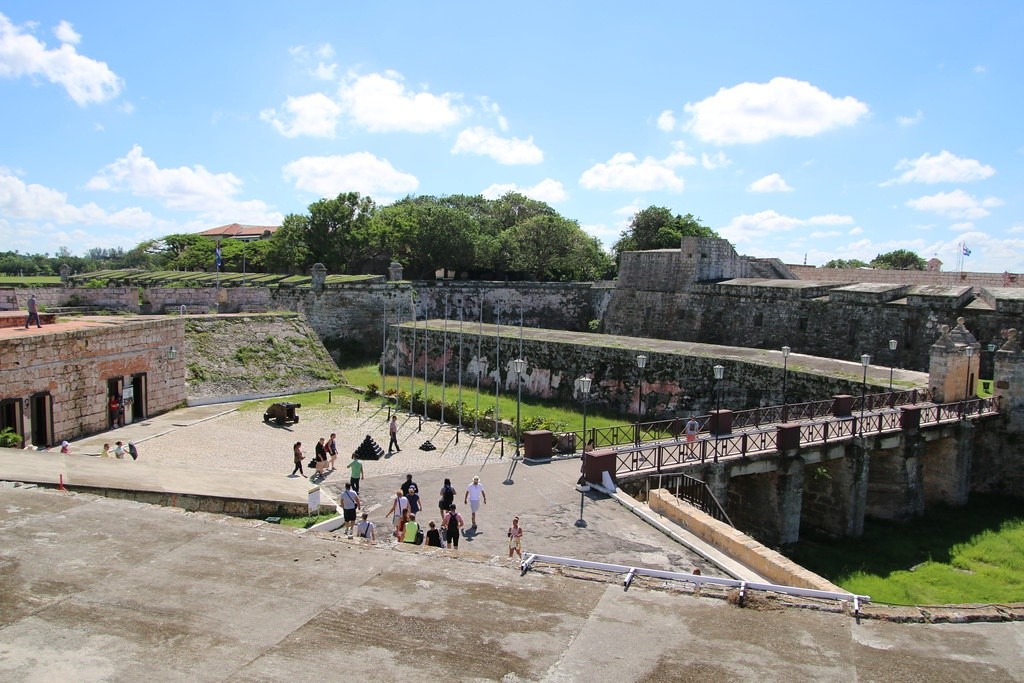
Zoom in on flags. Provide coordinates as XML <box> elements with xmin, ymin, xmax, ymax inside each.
<box><xmin>216</xmin><ymin>244</ymin><xmax>221</xmax><ymax>265</ymax></box>
<box><xmin>963</xmin><ymin>246</ymin><xmax>971</xmax><ymax>256</ymax></box>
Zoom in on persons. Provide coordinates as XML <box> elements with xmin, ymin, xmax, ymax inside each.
<box><xmin>386</xmin><ymin>475</ymin><xmax>422</xmax><ymax>543</ymax></box>
<box><xmin>293</xmin><ymin>442</ymin><xmax>307</xmax><ymax>478</ymax></box>
<box><xmin>443</xmin><ymin>504</ymin><xmax>463</xmax><ymax>549</ymax></box>
<box><xmin>684</xmin><ymin>415</ymin><xmax>699</xmax><ymax>455</ymax></box>
<box><xmin>347</xmin><ymin>454</ymin><xmax>363</xmax><ymax>494</ymax></box>
<box><xmin>425</xmin><ymin>521</ymin><xmax>441</xmax><ymax>547</ymax></box>
<box><xmin>25</xmin><ymin>441</ymin><xmax>68</xmax><ymax>452</ymax></box>
<box><xmin>439</xmin><ymin>478</ymin><xmax>456</xmax><ymax>519</ymax></box>
<box><xmin>464</xmin><ymin>476</ymin><xmax>486</xmax><ymax>527</ymax></box>
<box><xmin>356</xmin><ymin>513</ymin><xmax>375</xmax><ymax>540</ymax></box>
<box><xmin>327</xmin><ymin>433</ymin><xmax>338</xmax><ymax>470</ymax></box>
<box><xmin>110</xmin><ymin>395</ymin><xmax>124</xmax><ymax>428</ymax></box>
<box><xmin>341</xmin><ymin>484</ymin><xmax>360</xmax><ymax>534</ymax></box>
<box><xmin>101</xmin><ymin>444</ymin><xmax>110</xmax><ymax>457</ymax></box>
<box><xmin>389</xmin><ymin>416</ymin><xmax>402</xmax><ymax>451</ymax></box>
<box><xmin>115</xmin><ymin>441</ymin><xmax>129</xmax><ymax>459</ymax></box>
<box><xmin>585</xmin><ymin>438</ymin><xmax>594</xmax><ymax>452</ymax></box>
<box><xmin>25</xmin><ymin>294</ymin><xmax>42</xmax><ymax>328</ymax></box>
<box><xmin>311</xmin><ymin>438</ymin><xmax>327</xmax><ymax>480</ymax></box>
<box><xmin>509</xmin><ymin>517</ymin><xmax>522</xmax><ymax>557</ymax></box>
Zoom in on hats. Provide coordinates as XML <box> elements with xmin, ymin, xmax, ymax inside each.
<box><xmin>62</xmin><ymin>441</ymin><xmax>69</xmax><ymax>447</ymax></box>
<box><xmin>473</xmin><ymin>476</ymin><xmax>480</xmax><ymax>483</ymax></box>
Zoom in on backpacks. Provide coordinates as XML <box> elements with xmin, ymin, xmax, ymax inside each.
<box><xmin>448</xmin><ymin>512</ymin><xmax>458</xmax><ymax>532</ymax></box>
<box><xmin>443</xmin><ymin>486</ymin><xmax>453</xmax><ymax>505</ymax></box>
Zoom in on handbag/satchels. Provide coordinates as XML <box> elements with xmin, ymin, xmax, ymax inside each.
<box><xmin>416</xmin><ymin>524</ymin><xmax>424</xmax><ymax>545</ymax></box>
<box><xmin>438</xmin><ymin>528</ymin><xmax>446</xmax><ymax>547</ymax></box>
<box><xmin>355</xmin><ymin>503</ymin><xmax>358</xmax><ymax>509</ymax></box>
<box><xmin>324</xmin><ymin>440</ymin><xmax>333</xmax><ymax>452</ymax></box>
<box><xmin>360</xmin><ymin>534</ymin><xmax>366</xmax><ymax>537</ymax></box>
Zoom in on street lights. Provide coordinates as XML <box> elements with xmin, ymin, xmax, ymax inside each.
<box><xmin>888</xmin><ymin>339</ymin><xmax>898</xmax><ymax>409</ymax></box>
<box><xmin>961</xmin><ymin>344</ymin><xmax>974</xmax><ymax>420</ymax></box>
<box><xmin>781</xmin><ymin>346</ymin><xmax>791</xmax><ymax>423</ymax></box>
<box><xmin>636</xmin><ymin>354</ymin><xmax>647</xmax><ymax>445</ymax></box>
<box><xmin>987</xmin><ymin>343</ymin><xmax>996</xmax><ymax>380</ymax></box>
<box><xmin>576</xmin><ymin>376</ymin><xmax>593</xmax><ymax>493</ymax></box>
<box><xmin>513</xmin><ymin>357</ymin><xmax>525</xmax><ymax>460</ymax></box>
<box><xmin>713</xmin><ymin>364</ymin><xmax>725</xmax><ymax>463</ymax></box>
<box><xmin>859</xmin><ymin>353</ymin><xmax>872</xmax><ymax>437</ymax></box>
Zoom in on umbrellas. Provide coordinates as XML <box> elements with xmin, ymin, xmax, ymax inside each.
<box><xmin>129</xmin><ymin>443</ymin><xmax>138</xmax><ymax>460</ymax></box>
